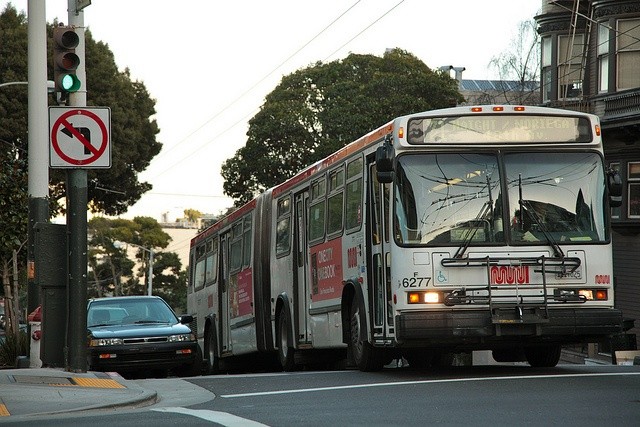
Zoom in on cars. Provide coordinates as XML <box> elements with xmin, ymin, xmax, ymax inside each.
<box><xmin>83</xmin><ymin>295</ymin><xmax>199</xmax><ymax>379</ymax></box>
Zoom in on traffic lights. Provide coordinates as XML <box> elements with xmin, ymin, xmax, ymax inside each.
<box><xmin>52</xmin><ymin>25</ymin><xmax>81</xmax><ymax>93</ymax></box>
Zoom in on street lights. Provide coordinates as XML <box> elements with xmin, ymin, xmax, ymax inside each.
<box><xmin>112</xmin><ymin>238</ymin><xmax>156</xmax><ymax>296</ymax></box>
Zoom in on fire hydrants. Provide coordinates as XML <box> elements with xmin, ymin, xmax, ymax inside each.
<box><xmin>27</xmin><ymin>303</ymin><xmax>43</xmax><ymax>369</ymax></box>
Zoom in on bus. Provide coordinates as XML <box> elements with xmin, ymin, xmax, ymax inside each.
<box><xmin>186</xmin><ymin>104</ymin><xmax>624</xmax><ymax>376</ymax></box>
